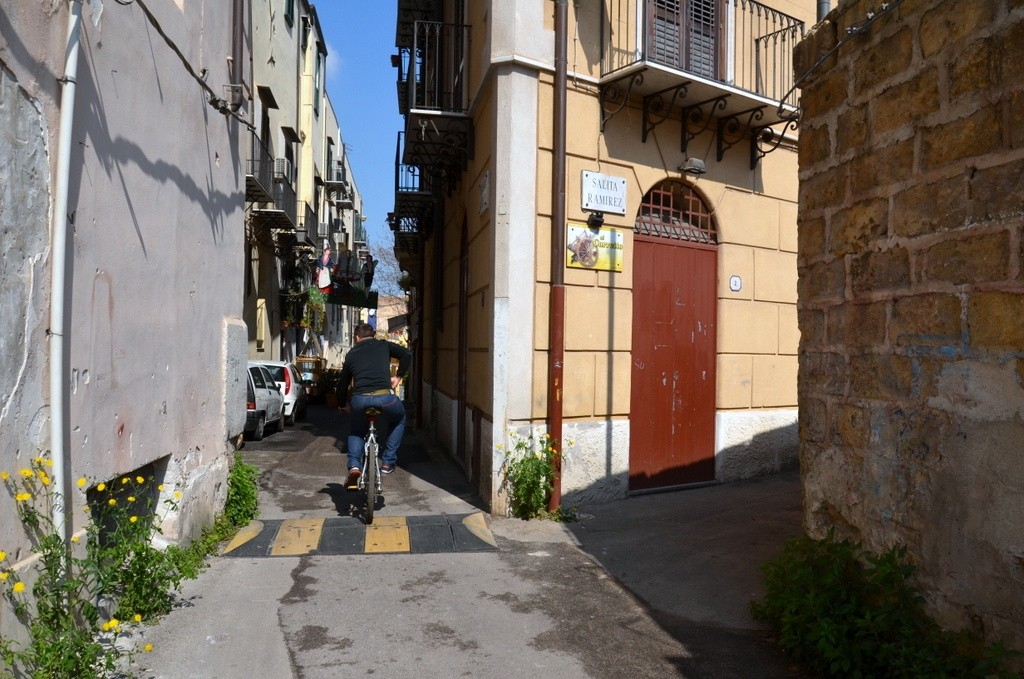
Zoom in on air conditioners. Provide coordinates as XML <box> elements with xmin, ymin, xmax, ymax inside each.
<box><xmin>335</xmin><ymin>233</ymin><xmax>346</xmax><ymax>244</ymax></box>
<box><xmin>276</xmin><ymin>158</ymin><xmax>290</xmax><ymax>180</ymax></box>
<box><xmin>318</xmin><ymin>222</ymin><xmax>329</xmax><ymax>237</ymax></box>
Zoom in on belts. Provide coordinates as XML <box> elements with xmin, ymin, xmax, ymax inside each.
<box><xmin>361</xmin><ymin>389</ymin><xmax>391</xmax><ymax>395</ymax></box>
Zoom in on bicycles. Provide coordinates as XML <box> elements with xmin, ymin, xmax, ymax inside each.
<box><xmin>347</xmin><ymin>408</ymin><xmax>382</xmax><ymax>525</ymax></box>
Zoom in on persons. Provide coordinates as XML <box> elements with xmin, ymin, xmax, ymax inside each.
<box><xmin>336</xmin><ymin>322</ymin><xmax>412</xmax><ymax>489</ymax></box>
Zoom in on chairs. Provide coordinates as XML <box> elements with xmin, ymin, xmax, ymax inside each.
<box><xmin>274</xmin><ymin>370</ymin><xmax>284</xmax><ymax>381</ymax></box>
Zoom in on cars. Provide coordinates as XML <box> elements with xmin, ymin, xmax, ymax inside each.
<box><xmin>242</xmin><ymin>363</ymin><xmax>285</xmax><ymax>441</ymax></box>
<box><xmin>250</xmin><ymin>361</ymin><xmax>319</xmax><ymax>425</ymax></box>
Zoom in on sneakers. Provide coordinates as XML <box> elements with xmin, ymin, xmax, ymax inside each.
<box><xmin>344</xmin><ymin>467</ymin><xmax>361</xmax><ymax>487</ymax></box>
<box><xmin>381</xmin><ymin>463</ymin><xmax>396</xmax><ymax>473</ymax></box>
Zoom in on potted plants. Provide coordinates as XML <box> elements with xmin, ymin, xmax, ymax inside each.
<box><xmin>283</xmin><ymin>313</ymin><xmax>294</xmax><ymax>327</ymax></box>
<box><xmin>325</xmin><ymin>376</ymin><xmax>338</xmax><ymax>408</ymax></box>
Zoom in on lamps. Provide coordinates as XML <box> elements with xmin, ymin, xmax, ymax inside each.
<box><xmin>678</xmin><ymin>157</ymin><xmax>707</xmax><ymax>174</ymax></box>
<box><xmin>296</xmin><ymin>222</ymin><xmax>308</xmax><ymax>244</ymax></box>
